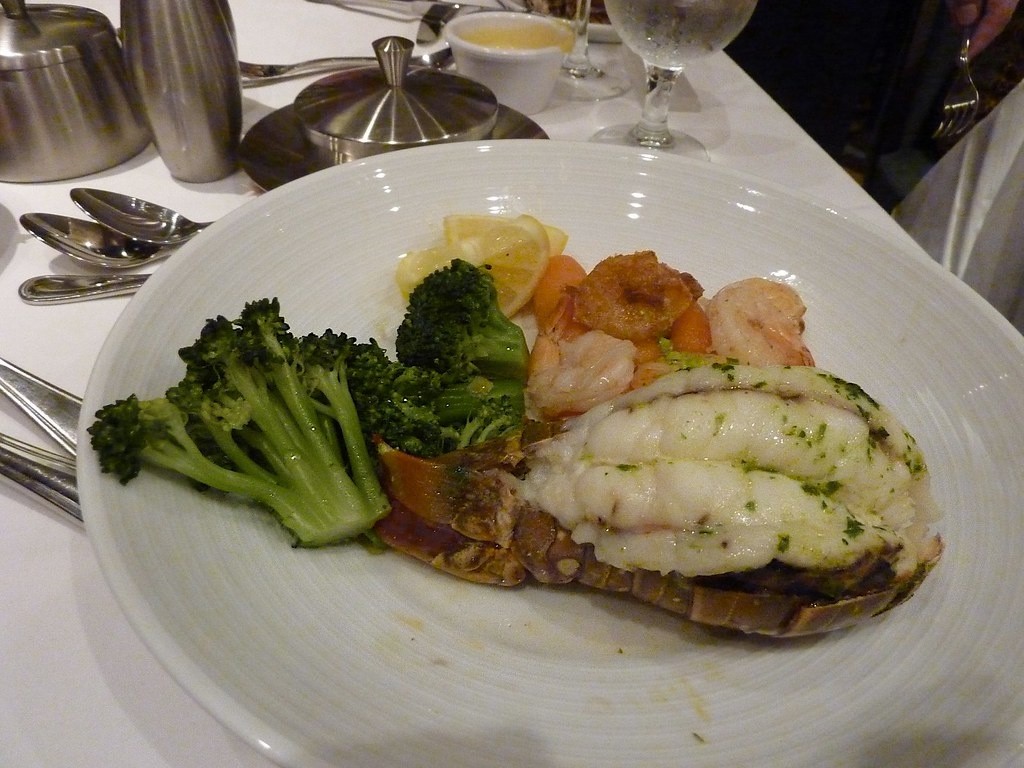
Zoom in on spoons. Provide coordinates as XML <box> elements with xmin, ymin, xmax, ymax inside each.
<box><xmin>19</xmin><ymin>212</ymin><xmax>184</xmax><ymax>269</ymax></box>
<box><xmin>69</xmin><ymin>188</ymin><xmax>215</xmax><ymax>245</ymax></box>
<box><xmin>238</xmin><ymin>48</ymin><xmax>455</xmax><ymax>88</ymax></box>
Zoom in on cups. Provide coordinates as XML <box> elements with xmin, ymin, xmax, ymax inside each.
<box><xmin>444</xmin><ymin>12</ymin><xmax>575</xmax><ymax>116</ymax></box>
<box><xmin>588</xmin><ymin>0</ymin><xmax>758</xmax><ymax>163</ymax></box>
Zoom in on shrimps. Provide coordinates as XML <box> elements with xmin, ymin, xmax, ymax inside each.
<box><xmin>365</xmin><ymin>358</ymin><xmax>945</xmax><ymax>641</ymax></box>
<box><xmin>522</xmin><ymin>249</ymin><xmax>817</xmax><ymax>417</ymax></box>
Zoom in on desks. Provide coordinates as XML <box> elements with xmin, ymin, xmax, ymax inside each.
<box><xmin>0</xmin><ymin>0</ymin><xmax>1024</xmax><ymax>768</ymax></box>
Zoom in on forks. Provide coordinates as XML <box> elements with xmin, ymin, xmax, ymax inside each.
<box><xmin>932</xmin><ymin>25</ymin><xmax>979</xmax><ymax>139</ymax></box>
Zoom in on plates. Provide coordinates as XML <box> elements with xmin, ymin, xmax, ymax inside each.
<box><xmin>75</xmin><ymin>135</ymin><xmax>1024</xmax><ymax>768</ymax></box>
<box><xmin>237</xmin><ymin>102</ymin><xmax>550</xmax><ymax>193</ymax></box>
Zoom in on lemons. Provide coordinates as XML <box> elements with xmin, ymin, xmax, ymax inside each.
<box><xmin>395</xmin><ymin>213</ymin><xmax>570</xmax><ymax>318</ymax></box>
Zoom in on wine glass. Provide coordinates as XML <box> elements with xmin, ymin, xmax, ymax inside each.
<box><xmin>554</xmin><ymin>0</ymin><xmax>633</xmax><ymax>103</ymax></box>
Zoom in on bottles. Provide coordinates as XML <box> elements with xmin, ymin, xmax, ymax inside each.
<box><xmin>120</xmin><ymin>0</ymin><xmax>245</xmax><ymax>183</ymax></box>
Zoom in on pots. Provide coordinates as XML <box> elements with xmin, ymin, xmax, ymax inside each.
<box><xmin>292</xmin><ymin>35</ymin><xmax>499</xmax><ymax>171</ymax></box>
<box><xmin>0</xmin><ymin>0</ymin><xmax>151</xmax><ymax>183</ymax></box>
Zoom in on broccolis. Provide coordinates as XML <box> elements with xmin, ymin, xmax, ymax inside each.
<box><xmin>86</xmin><ymin>257</ymin><xmax>533</xmax><ymax>550</ymax></box>
